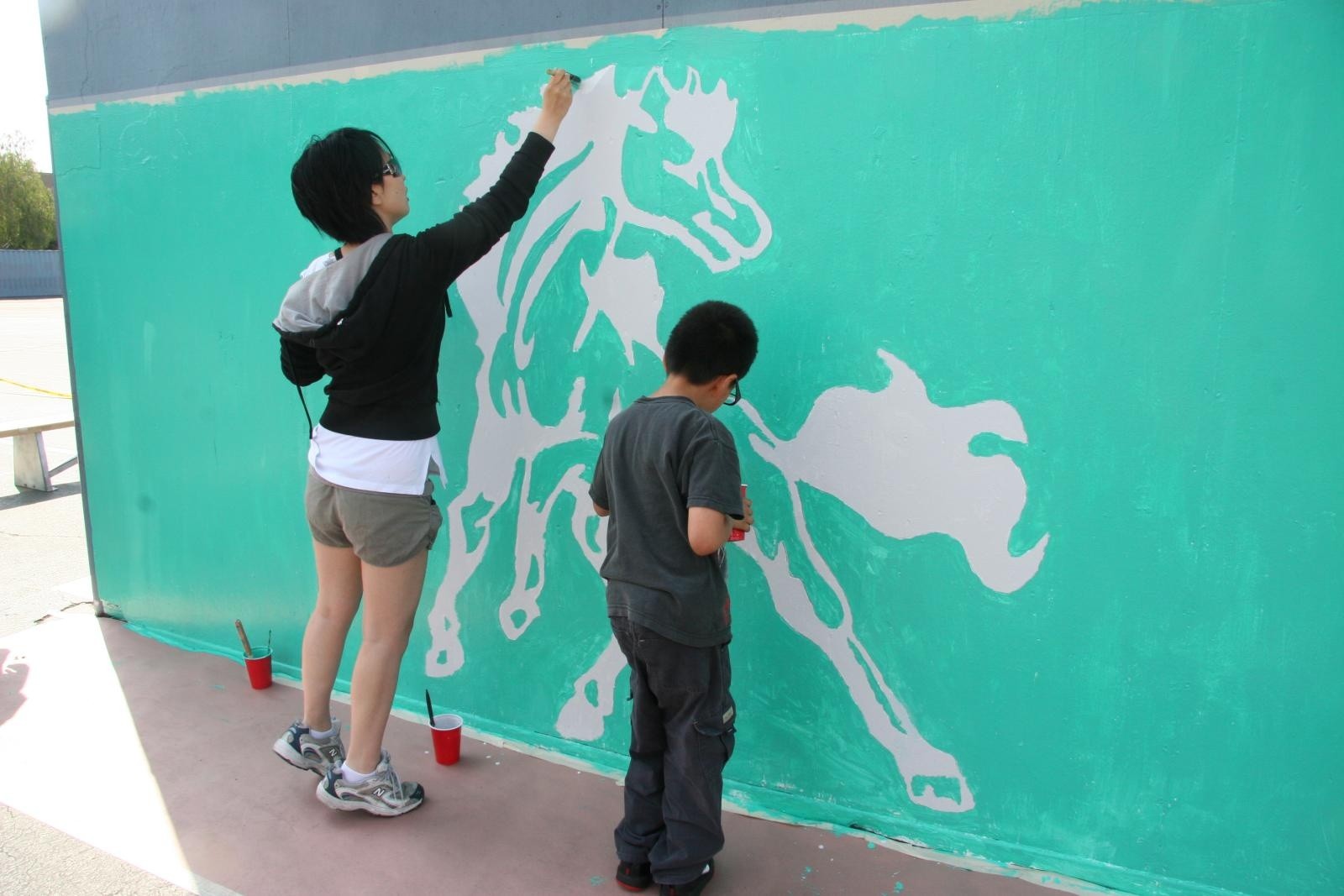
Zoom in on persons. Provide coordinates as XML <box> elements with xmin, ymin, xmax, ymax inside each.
<box><xmin>588</xmin><ymin>301</ymin><xmax>759</xmax><ymax>896</ymax></box>
<box><xmin>270</xmin><ymin>67</ymin><xmax>574</xmax><ymax>816</ymax></box>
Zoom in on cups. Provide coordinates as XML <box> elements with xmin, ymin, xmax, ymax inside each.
<box><xmin>429</xmin><ymin>714</ymin><xmax>463</xmax><ymax>766</ymax></box>
<box><xmin>729</xmin><ymin>484</ymin><xmax>748</xmax><ymax>542</ymax></box>
<box><xmin>243</xmin><ymin>647</ymin><xmax>273</xmax><ymax>689</ymax></box>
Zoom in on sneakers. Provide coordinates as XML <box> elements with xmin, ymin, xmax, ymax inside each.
<box><xmin>660</xmin><ymin>857</ymin><xmax>715</xmax><ymax>896</ymax></box>
<box><xmin>615</xmin><ymin>859</ymin><xmax>654</xmax><ymax>892</ymax></box>
<box><xmin>271</xmin><ymin>715</ymin><xmax>347</xmax><ymax>777</ymax></box>
<box><xmin>315</xmin><ymin>750</ymin><xmax>425</xmax><ymax>817</ymax></box>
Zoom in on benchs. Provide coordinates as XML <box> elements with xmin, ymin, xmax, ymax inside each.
<box><xmin>0</xmin><ymin>420</ymin><xmax>76</xmax><ymax>492</ymax></box>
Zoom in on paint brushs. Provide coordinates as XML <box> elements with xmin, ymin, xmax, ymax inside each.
<box><xmin>424</xmin><ymin>688</ymin><xmax>437</xmax><ymax>727</ymax></box>
<box><xmin>268</xmin><ymin>631</ymin><xmax>272</xmax><ymax>654</ymax></box>
<box><xmin>547</xmin><ymin>66</ymin><xmax>580</xmax><ymax>82</ymax></box>
<box><xmin>235</xmin><ymin>619</ymin><xmax>254</xmax><ymax>659</ymax></box>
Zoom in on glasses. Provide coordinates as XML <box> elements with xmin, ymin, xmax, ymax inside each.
<box><xmin>724</xmin><ymin>369</ymin><xmax>742</xmax><ymax>406</ymax></box>
<box><xmin>380</xmin><ymin>158</ymin><xmax>402</xmax><ymax>178</ymax></box>
